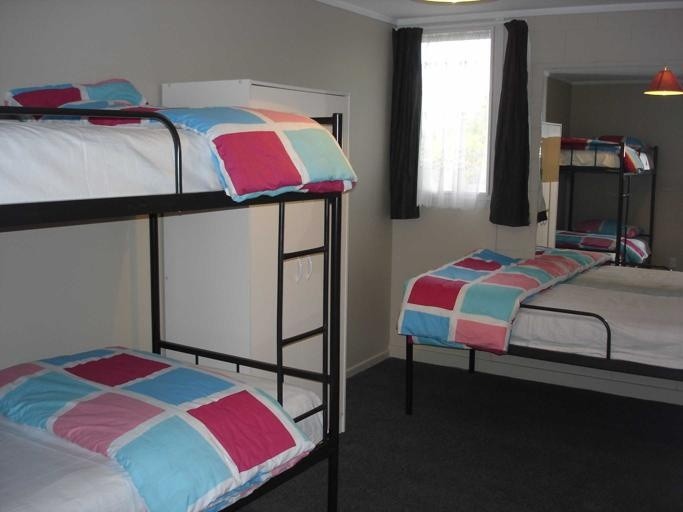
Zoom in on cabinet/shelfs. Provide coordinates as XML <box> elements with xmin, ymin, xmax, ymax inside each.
<box><xmin>161</xmin><ymin>78</ymin><xmax>351</xmax><ymax>434</ymax></box>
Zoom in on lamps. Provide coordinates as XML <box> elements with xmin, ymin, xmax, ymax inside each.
<box><xmin>643</xmin><ymin>65</ymin><xmax>683</xmax><ymax>96</ymax></box>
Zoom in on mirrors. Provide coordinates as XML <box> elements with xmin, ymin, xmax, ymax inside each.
<box><xmin>535</xmin><ymin>74</ymin><xmax>683</xmax><ymax>271</ymax></box>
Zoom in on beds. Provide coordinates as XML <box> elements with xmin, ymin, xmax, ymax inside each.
<box><xmin>534</xmin><ymin>248</ymin><xmax>682</xmax><ymax>297</ymax></box>
<box><xmin>0</xmin><ymin>105</ymin><xmax>342</xmax><ymax>511</ymax></box>
<box><xmin>396</xmin><ymin>247</ymin><xmax>683</xmax><ymax>416</ymax></box>
<box><xmin>561</xmin><ymin>134</ymin><xmax>658</xmax><ymax>268</ymax></box>
